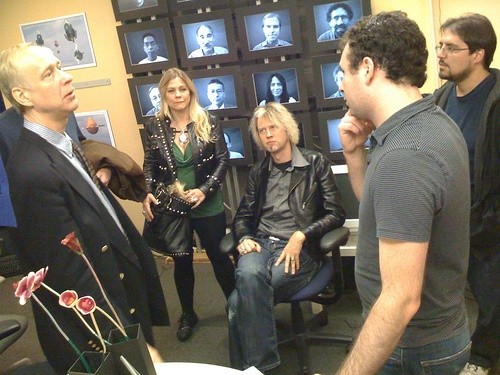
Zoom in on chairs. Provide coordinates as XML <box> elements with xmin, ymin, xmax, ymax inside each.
<box><xmin>220</xmin><ymin>225</ymin><xmax>353</xmax><ymax>375</ymax></box>
<box><xmin>0</xmin><ymin>315</ymin><xmax>28</xmax><ymax>355</ymax></box>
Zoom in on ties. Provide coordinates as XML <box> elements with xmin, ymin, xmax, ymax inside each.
<box><xmin>70</xmin><ymin>140</ymin><xmax>103</xmax><ymax>191</ymax></box>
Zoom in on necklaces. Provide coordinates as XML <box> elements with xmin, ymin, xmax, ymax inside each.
<box><xmin>170</xmin><ymin>111</ymin><xmax>188</xmax><ymax>143</ymax></box>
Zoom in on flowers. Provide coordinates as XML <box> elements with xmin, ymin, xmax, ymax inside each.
<box><xmin>13</xmin><ymin>232</ymin><xmax>128</xmax><ymax>373</ymax></box>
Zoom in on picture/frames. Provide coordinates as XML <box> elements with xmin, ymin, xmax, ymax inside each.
<box><xmin>74</xmin><ymin>110</ymin><xmax>116</xmax><ymax>148</ymax></box>
<box><xmin>110</xmin><ymin>0</ymin><xmax>373</xmax><ymax>169</ymax></box>
<box><xmin>18</xmin><ymin>12</ymin><xmax>97</xmax><ymax>71</ymax></box>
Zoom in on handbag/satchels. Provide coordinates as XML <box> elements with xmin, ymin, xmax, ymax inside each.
<box><xmin>142</xmin><ymin>182</ymin><xmax>194</xmax><ymax>258</ymax></box>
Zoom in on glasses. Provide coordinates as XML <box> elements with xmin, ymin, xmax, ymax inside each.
<box><xmin>435</xmin><ymin>44</ymin><xmax>471</xmax><ymax>53</ymax></box>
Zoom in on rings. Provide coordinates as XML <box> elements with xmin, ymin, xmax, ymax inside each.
<box><xmin>241</xmin><ymin>249</ymin><xmax>245</xmax><ymax>253</ymax></box>
<box><xmin>191</xmin><ymin>195</ymin><xmax>197</xmax><ymax>202</ymax></box>
<box><xmin>290</xmin><ymin>258</ymin><xmax>295</xmax><ymax>261</ymax></box>
<box><xmin>142</xmin><ymin>211</ymin><xmax>146</xmax><ymax>213</ymax></box>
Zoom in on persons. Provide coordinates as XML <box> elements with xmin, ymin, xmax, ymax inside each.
<box><xmin>258</xmin><ymin>72</ymin><xmax>296</xmax><ymax>106</ymax></box>
<box><xmin>432</xmin><ymin>12</ymin><xmax>500</xmax><ymax>375</ymax></box>
<box><xmin>330</xmin><ymin>65</ymin><xmax>344</xmax><ymax>97</ymax></box>
<box><xmin>317</xmin><ymin>3</ymin><xmax>356</xmax><ymax>42</ymax></box>
<box><xmin>187</xmin><ymin>23</ymin><xmax>228</xmax><ymax>58</ymax></box>
<box><xmin>141</xmin><ymin>68</ymin><xmax>236</xmax><ymax>340</ymax></box>
<box><xmin>203</xmin><ymin>79</ymin><xmax>236</xmax><ymax>109</ymax></box>
<box><xmin>0</xmin><ymin>44</ymin><xmax>169</xmax><ymax>375</ymax></box>
<box><xmin>138</xmin><ymin>33</ymin><xmax>169</xmax><ymax>64</ymax></box>
<box><xmin>224</xmin><ymin>133</ymin><xmax>244</xmax><ymax>159</ymax></box>
<box><xmin>145</xmin><ymin>86</ymin><xmax>163</xmax><ymax>117</ymax></box>
<box><xmin>226</xmin><ymin>102</ymin><xmax>346</xmax><ymax>375</ymax></box>
<box><xmin>335</xmin><ymin>10</ymin><xmax>471</xmax><ymax>375</ymax></box>
<box><xmin>252</xmin><ymin>14</ymin><xmax>291</xmax><ymax>51</ymax></box>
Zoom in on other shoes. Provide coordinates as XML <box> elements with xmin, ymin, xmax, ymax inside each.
<box><xmin>176</xmin><ymin>311</ymin><xmax>198</xmax><ymax>341</ymax></box>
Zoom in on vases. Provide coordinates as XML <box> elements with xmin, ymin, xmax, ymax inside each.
<box><xmin>68</xmin><ymin>324</ymin><xmax>157</xmax><ymax>375</ymax></box>
